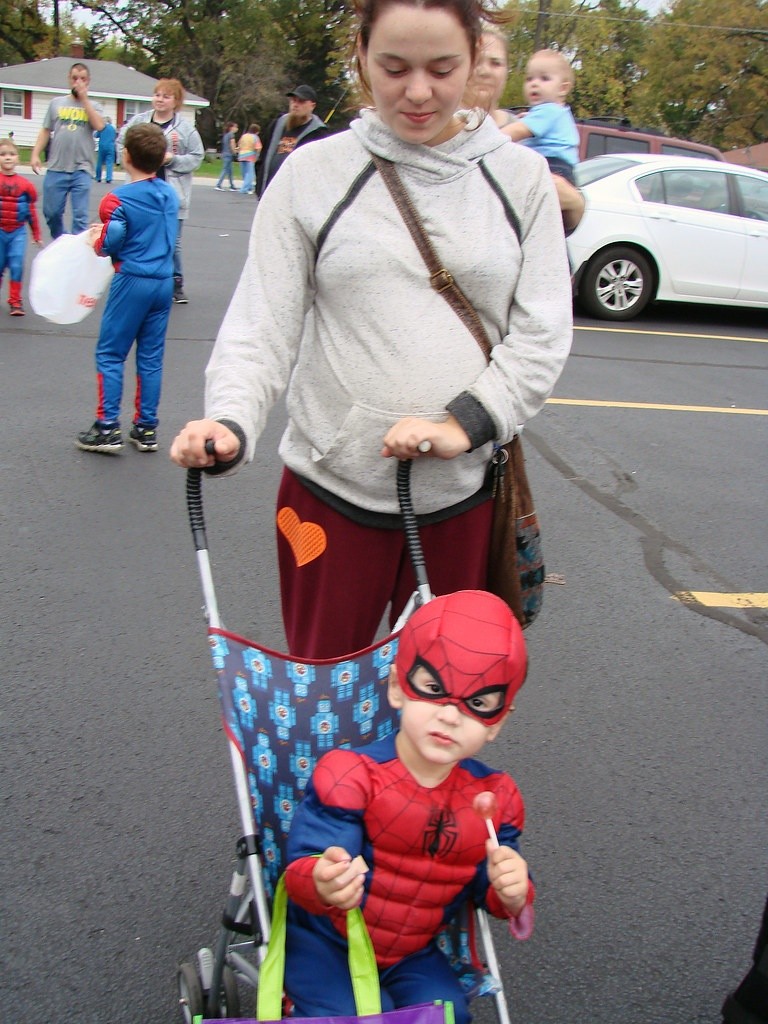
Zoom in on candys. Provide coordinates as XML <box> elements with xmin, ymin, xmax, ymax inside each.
<box><xmin>472</xmin><ymin>790</ymin><xmax>498</xmax><ymax>819</ymax></box>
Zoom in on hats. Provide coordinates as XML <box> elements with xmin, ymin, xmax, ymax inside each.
<box><xmin>285</xmin><ymin>84</ymin><xmax>319</xmax><ymax>104</ymax></box>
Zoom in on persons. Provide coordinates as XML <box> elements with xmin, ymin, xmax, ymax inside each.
<box><xmin>255</xmin><ymin>85</ymin><xmax>332</xmax><ymax>201</ymax></box>
<box><xmin>73</xmin><ymin>123</ymin><xmax>180</xmax><ymax>450</ymax></box>
<box><xmin>500</xmin><ymin>49</ymin><xmax>579</xmax><ymax>188</ymax></box>
<box><xmin>8</xmin><ymin>132</ymin><xmax>14</xmax><ymax>141</ymax></box>
<box><xmin>0</xmin><ymin>138</ymin><xmax>46</xmax><ymax>316</ymax></box>
<box><xmin>114</xmin><ymin>77</ymin><xmax>205</xmax><ymax>303</ymax></box>
<box><xmin>94</xmin><ymin>116</ymin><xmax>116</xmax><ymax>183</ymax></box>
<box><xmin>272</xmin><ymin>590</ymin><xmax>536</xmax><ymax>1023</ymax></box>
<box><xmin>213</xmin><ymin>122</ymin><xmax>239</xmax><ymax>191</ymax></box>
<box><xmin>169</xmin><ymin>0</ymin><xmax>574</xmax><ymax>655</ymax></box>
<box><xmin>462</xmin><ymin>17</ymin><xmax>586</xmax><ymax>238</ymax></box>
<box><xmin>30</xmin><ymin>63</ymin><xmax>106</xmax><ymax>241</ymax></box>
<box><xmin>237</xmin><ymin>124</ymin><xmax>263</xmax><ymax>195</ymax></box>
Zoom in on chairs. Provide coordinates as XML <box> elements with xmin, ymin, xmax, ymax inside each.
<box><xmin>659</xmin><ymin>174</ymin><xmax>726</xmax><ymax>209</ymax></box>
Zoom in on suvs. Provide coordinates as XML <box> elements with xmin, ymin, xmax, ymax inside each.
<box><xmin>499</xmin><ymin>101</ymin><xmax>729</xmax><ymax>189</ymax></box>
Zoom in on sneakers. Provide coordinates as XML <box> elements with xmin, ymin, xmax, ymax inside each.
<box><xmin>128</xmin><ymin>424</ymin><xmax>159</xmax><ymax>451</ymax></box>
<box><xmin>10</xmin><ymin>303</ymin><xmax>26</xmax><ymax>315</ymax></box>
<box><xmin>73</xmin><ymin>421</ymin><xmax>125</xmax><ymax>453</ymax></box>
<box><xmin>172</xmin><ymin>288</ymin><xmax>189</xmax><ymax>304</ymax></box>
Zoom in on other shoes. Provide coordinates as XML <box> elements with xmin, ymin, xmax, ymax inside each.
<box><xmin>248</xmin><ymin>191</ymin><xmax>252</xmax><ymax>194</ymax></box>
<box><xmin>214</xmin><ymin>187</ymin><xmax>224</xmax><ymax>191</ymax></box>
<box><xmin>230</xmin><ymin>187</ymin><xmax>237</xmax><ymax>191</ymax></box>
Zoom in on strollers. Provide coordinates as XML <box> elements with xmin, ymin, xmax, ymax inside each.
<box><xmin>185</xmin><ymin>437</ymin><xmax>519</xmax><ymax>1024</ymax></box>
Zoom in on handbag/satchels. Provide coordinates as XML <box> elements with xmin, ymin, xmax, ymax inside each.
<box><xmin>493</xmin><ymin>431</ymin><xmax>547</xmax><ymax>630</ymax></box>
<box><xmin>194</xmin><ymin>851</ymin><xmax>455</xmax><ymax>1024</ymax></box>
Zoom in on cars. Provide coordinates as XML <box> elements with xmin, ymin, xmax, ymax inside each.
<box><xmin>560</xmin><ymin>149</ymin><xmax>768</xmax><ymax>326</ymax></box>
